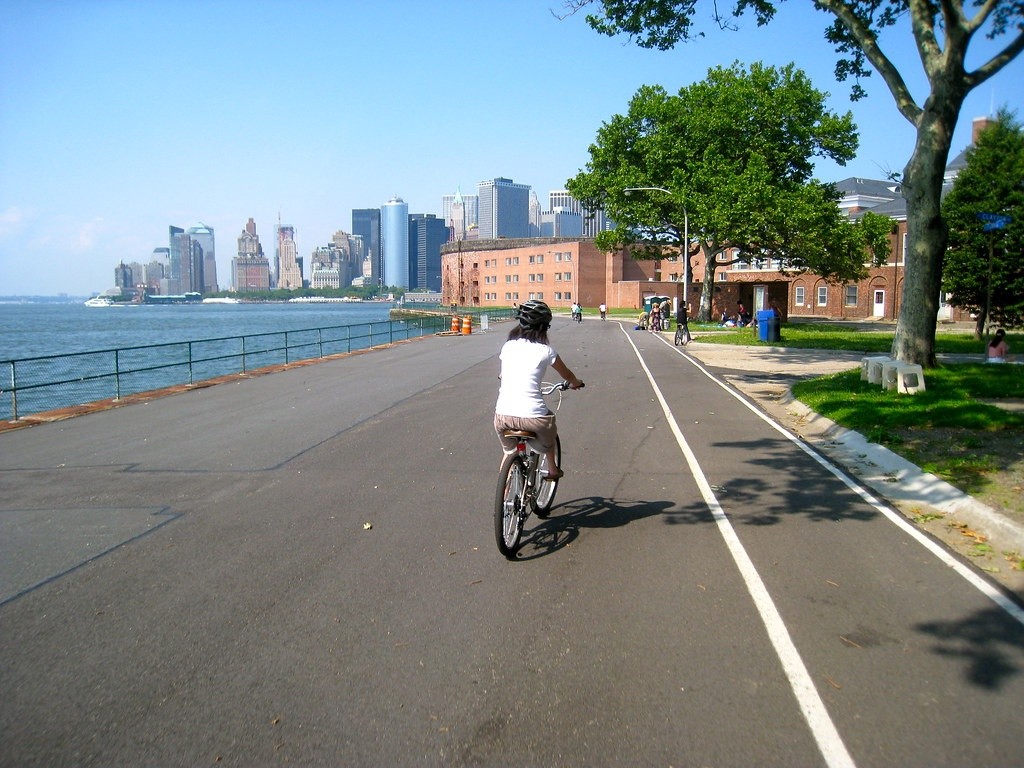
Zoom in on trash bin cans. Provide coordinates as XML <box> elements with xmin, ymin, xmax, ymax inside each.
<box><xmin>756</xmin><ymin>310</ymin><xmax>781</xmax><ymax>343</ymax></box>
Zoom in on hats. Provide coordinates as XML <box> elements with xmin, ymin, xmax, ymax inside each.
<box><xmin>667</xmin><ymin>300</ymin><xmax>671</xmax><ymax>305</ymax></box>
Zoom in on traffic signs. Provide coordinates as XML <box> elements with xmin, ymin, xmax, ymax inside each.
<box><xmin>974</xmin><ymin>212</ymin><xmax>1013</xmax><ymax>237</ymax></box>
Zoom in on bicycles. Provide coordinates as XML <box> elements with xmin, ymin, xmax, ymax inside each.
<box><xmin>493</xmin><ymin>380</ymin><xmax>585</xmax><ymax>557</ymax></box>
<box><xmin>675</xmin><ymin>316</ymin><xmax>688</xmax><ymax>346</ymax></box>
<box><xmin>572</xmin><ymin>313</ymin><xmax>581</xmax><ymax>324</ymax></box>
<box><xmin>601</xmin><ymin>312</ymin><xmax>606</xmax><ymax>321</ymax></box>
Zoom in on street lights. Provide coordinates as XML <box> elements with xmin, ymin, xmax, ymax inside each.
<box><xmin>623</xmin><ymin>186</ymin><xmax>689</xmax><ymax>310</ymax></box>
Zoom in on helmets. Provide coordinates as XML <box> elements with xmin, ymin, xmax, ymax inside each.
<box><xmin>515</xmin><ymin>300</ymin><xmax>552</xmax><ymax>330</ymax></box>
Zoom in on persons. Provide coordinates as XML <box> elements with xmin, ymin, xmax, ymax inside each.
<box><xmin>493</xmin><ymin>299</ymin><xmax>583</xmax><ymax>518</ymax></box>
<box><xmin>638</xmin><ymin>300</ymin><xmax>672</xmax><ymax>333</ymax></box>
<box><xmin>512</xmin><ymin>303</ymin><xmax>517</xmax><ymax>313</ymax></box>
<box><xmin>598</xmin><ymin>302</ymin><xmax>606</xmax><ymax>322</ymax></box>
<box><xmin>676</xmin><ymin>300</ymin><xmax>694</xmax><ymax>343</ymax></box>
<box><xmin>571</xmin><ymin>302</ymin><xmax>583</xmax><ymax>324</ymax></box>
<box><xmin>738</xmin><ymin>304</ymin><xmax>747</xmax><ymax>326</ymax></box>
<box><xmin>988</xmin><ymin>329</ymin><xmax>1017</xmax><ymax>363</ymax></box>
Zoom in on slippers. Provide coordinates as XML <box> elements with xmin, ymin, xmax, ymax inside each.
<box><xmin>546</xmin><ymin>466</ymin><xmax>563</xmax><ymax>481</ymax></box>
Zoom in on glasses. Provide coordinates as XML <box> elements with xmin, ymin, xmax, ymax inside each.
<box><xmin>546</xmin><ymin>325</ymin><xmax>551</xmax><ymax>329</ymax></box>
